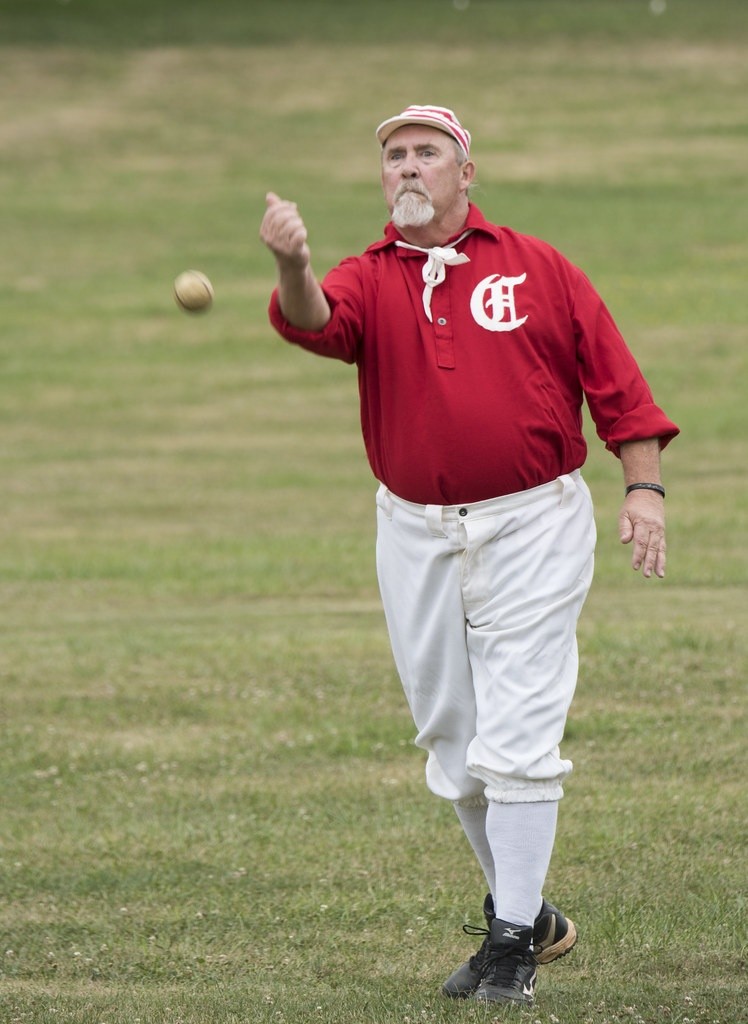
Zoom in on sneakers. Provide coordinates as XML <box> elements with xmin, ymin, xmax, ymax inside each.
<box><xmin>471</xmin><ymin>917</ymin><xmax>543</xmax><ymax>1003</ymax></box>
<box><xmin>442</xmin><ymin>892</ymin><xmax>576</xmax><ymax>999</ymax></box>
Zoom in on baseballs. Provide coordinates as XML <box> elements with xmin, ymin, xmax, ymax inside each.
<box><xmin>172</xmin><ymin>268</ymin><xmax>215</xmax><ymax>312</ymax></box>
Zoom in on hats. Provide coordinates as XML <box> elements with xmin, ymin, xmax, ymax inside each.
<box><xmin>375</xmin><ymin>104</ymin><xmax>471</xmax><ymax>160</ymax></box>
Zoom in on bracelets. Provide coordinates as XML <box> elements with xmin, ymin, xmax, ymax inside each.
<box><xmin>625</xmin><ymin>483</ymin><xmax>665</xmax><ymax>499</ymax></box>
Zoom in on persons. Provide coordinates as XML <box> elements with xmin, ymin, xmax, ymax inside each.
<box><xmin>259</xmin><ymin>105</ymin><xmax>681</xmax><ymax>1010</ymax></box>
